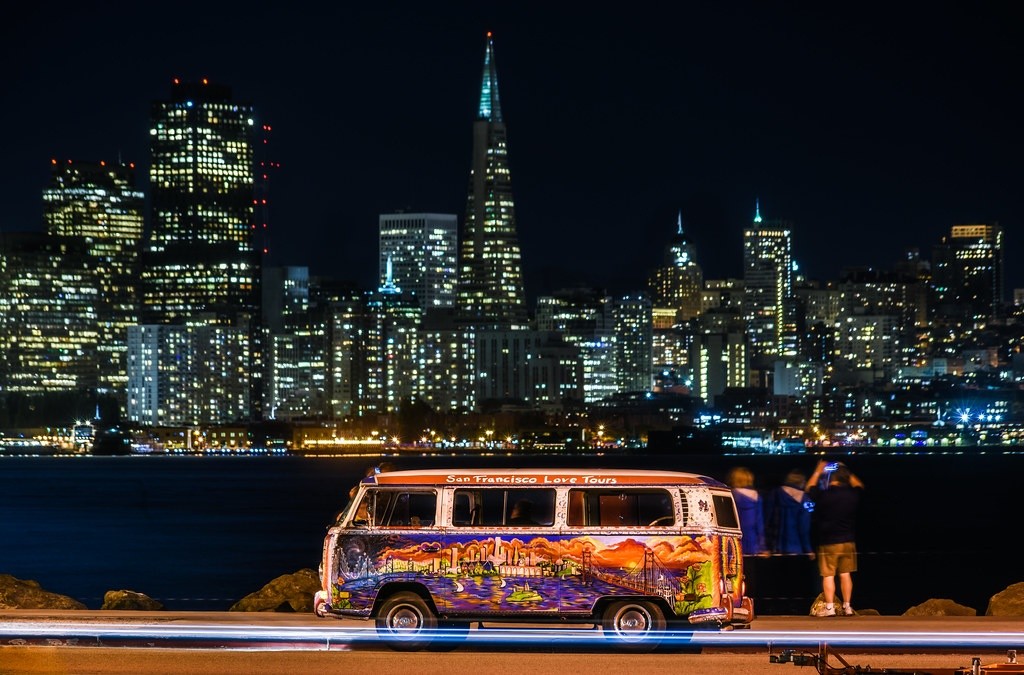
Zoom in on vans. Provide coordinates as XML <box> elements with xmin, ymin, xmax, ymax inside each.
<box><xmin>311</xmin><ymin>465</ymin><xmax>756</xmax><ymax>656</ymax></box>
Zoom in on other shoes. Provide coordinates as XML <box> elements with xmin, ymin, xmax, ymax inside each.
<box><xmin>843</xmin><ymin>605</ymin><xmax>852</xmax><ymax>614</ymax></box>
<box><xmin>824</xmin><ymin>605</ymin><xmax>836</xmax><ymax>616</ymax></box>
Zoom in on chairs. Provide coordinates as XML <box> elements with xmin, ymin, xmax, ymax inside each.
<box><xmin>470</xmin><ymin>504</ymin><xmax>480</xmax><ymax>525</ymax></box>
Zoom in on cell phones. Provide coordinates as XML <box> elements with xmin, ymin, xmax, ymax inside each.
<box><xmin>825</xmin><ymin>462</ymin><xmax>839</xmax><ymax>471</ymax></box>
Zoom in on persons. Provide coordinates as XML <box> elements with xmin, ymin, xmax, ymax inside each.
<box><xmin>336</xmin><ymin>462</ymin><xmax>397</xmax><ymax>524</ymax></box>
<box><xmin>508</xmin><ymin>499</ymin><xmax>539</xmax><ymax>525</ymax></box>
<box><xmin>729</xmin><ymin>458</ymin><xmax>865</xmax><ymax>616</ymax></box>
